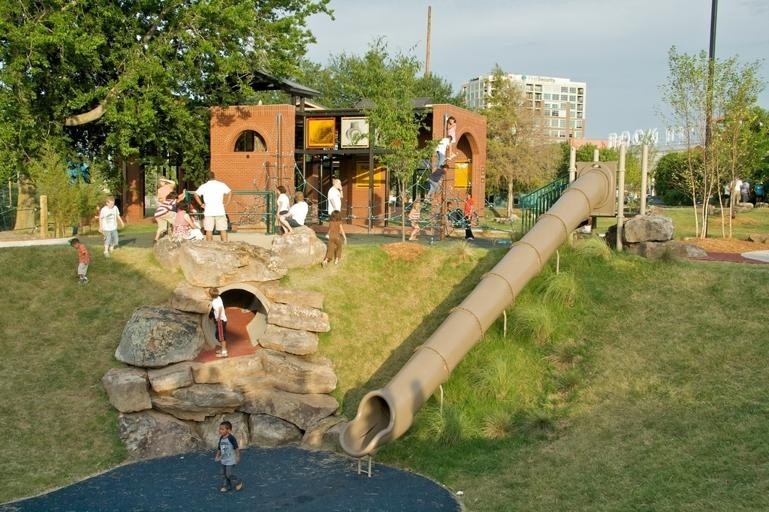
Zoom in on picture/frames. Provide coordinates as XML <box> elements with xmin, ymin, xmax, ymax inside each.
<box><xmin>306</xmin><ymin>117</ymin><xmax>336</xmax><ymax>149</ymax></box>
<box><xmin>341</xmin><ymin>117</ymin><xmax>369</xmax><ymax>149</ymax></box>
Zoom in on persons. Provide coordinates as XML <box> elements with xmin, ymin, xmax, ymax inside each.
<box><xmin>209</xmin><ymin>286</ymin><xmax>229</xmax><ymax>359</ymax></box>
<box><xmin>465</xmin><ymin>191</ymin><xmax>474</xmax><ymax>229</ymax></box>
<box><xmin>215</xmin><ymin>422</ymin><xmax>243</xmax><ymax>492</ymax></box>
<box><xmin>423</xmin><ymin>164</ymin><xmax>449</xmax><ymax>203</ymax></box>
<box><xmin>435</xmin><ymin>136</ymin><xmax>452</xmax><ymax>168</ymax></box>
<box><xmin>325</xmin><ymin>178</ymin><xmax>344</xmax><ymax>240</ymax></box>
<box><xmin>154</xmin><ymin>171</ymin><xmax>232</xmax><ymax>242</ymax></box>
<box><xmin>723</xmin><ymin>177</ymin><xmax>765</xmax><ymax>208</ymax></box>
<box><xmin>275</xmin><ymin>186</ymin><xmax>290</xmax><ymax>235</ymax></box>
<box><xmin>280</xmin><ymin>191</ymin><xmax>309</xmax><ymax>235</ymax></box>
<box><xmin>446</xmin><ymin>202</ymin><xmax>474</xmax><ymax>240</ymax></box>
<box><xmin>69</xmin><ymin>237</ymin><xmax>89</xmax><ymax>284</ymax></box>
<box><xmin>98</xmin><ymin>196</ymin><xmax>124</xmax><ymax>257</ymax></box>
<box><xmin>321</xmin><ymin>210</ymin><xmax>347</xmax><ymax>268</ymax></box>
<box><xmin>408</xmin><ymin>197</ymin><xmax>422</xmax><ymax>241</ymax></box>
<box><xmin>447</xmin><ymin>117</ymin><xmax>457</xmax><ymax>160</ymax></box>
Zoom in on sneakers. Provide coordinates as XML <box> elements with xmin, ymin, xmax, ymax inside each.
<box><xmin>216</xmin><ymin>350</ymin><xmax>222</xmax><ymax>353</ymax></box>
<box><xmin>236</xmin><ymin>481</ymin><xmax>242</xmax><ymax>490</ymax></box>
<box><xmin>216</xmin><ymin>352</ymin><xmax>228</xmax><ymax>357</ymax></box>
<box><xmin>221</xmin><ymin>487</ymin><xmax>228</xmax><ymax>492</ymax></box>
